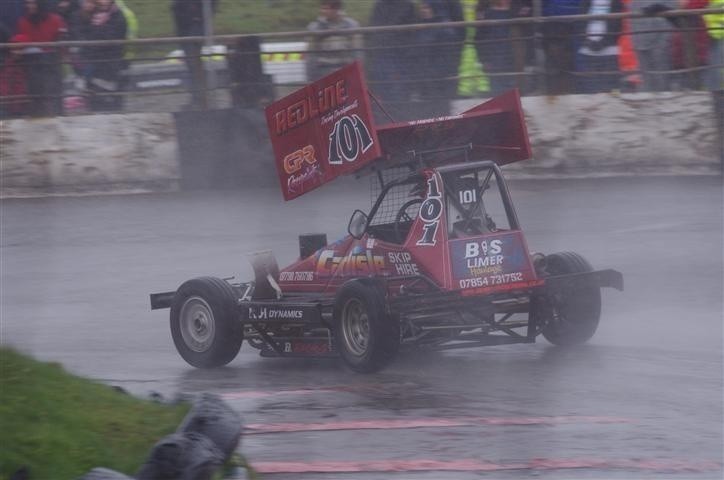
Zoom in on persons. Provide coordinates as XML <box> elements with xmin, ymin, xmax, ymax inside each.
<box><xmin>306</xmin><ymin>1</ymin><xmax>362</xmax><ymax>81</ymax></box>
<box><xmin>0</xmin><ymin>1</ymin><xmax>139</xmax><ymax>118</ymax></box>
<box><xmin>363</xmin><ymin>1</ymin><xmax>723</xmax><ymax>118</ymax></box>
<box><xmin>76</xmin><ymin>430</ymin><xmax>219</xmax><ymax>480</ymax></box>
<box><xmin>173</xmin><ymin>395</ymin><xmax>251</xmax><ymax>480</ymax></box>
